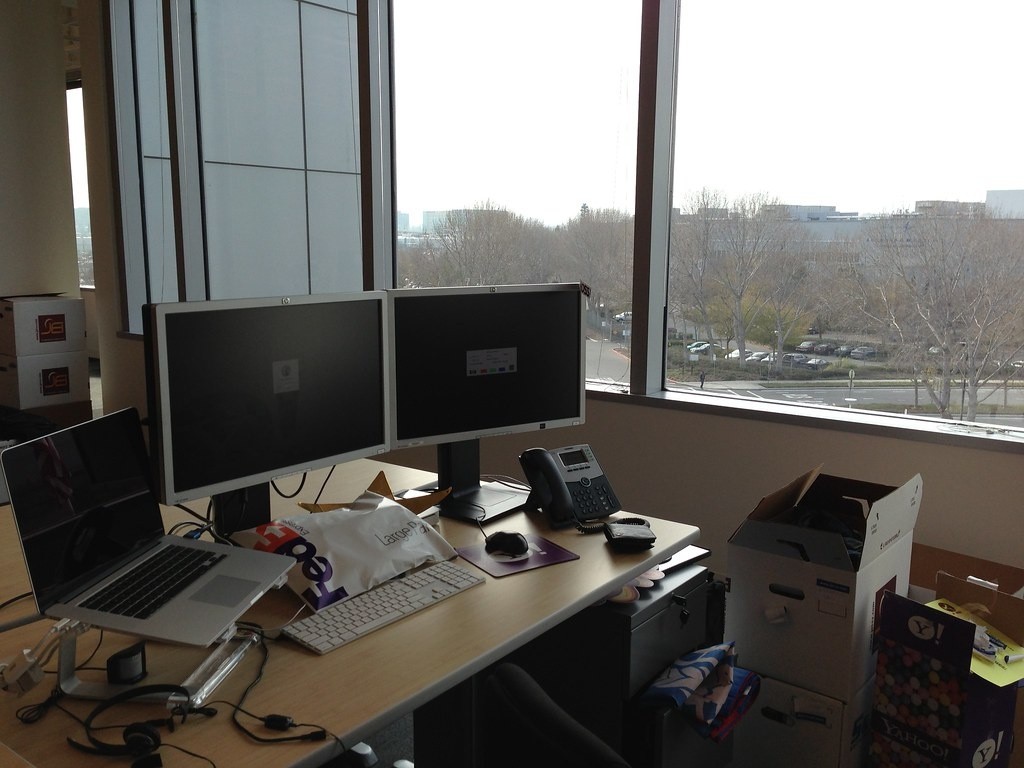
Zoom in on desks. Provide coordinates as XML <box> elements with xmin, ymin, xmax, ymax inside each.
<box><xmin>0</xmin><ymin>461</ymin><xmax>702</xmax><ymax>768</ymax></box>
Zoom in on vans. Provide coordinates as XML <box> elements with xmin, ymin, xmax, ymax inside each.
<box><xmin>928</xmin><ymin>341</ymin><xmax>966</xmax><ymax>354</ymax></box>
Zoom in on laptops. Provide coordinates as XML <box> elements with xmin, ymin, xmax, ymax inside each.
<box><xmin>0</xmin><ymin>406</ymin><xmax>297</xmax><ymax>650</ymax></box>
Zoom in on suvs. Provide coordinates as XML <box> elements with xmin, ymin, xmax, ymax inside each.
<box><xmin>668</xmin><ymin>332</ymin><xmax>692</xmax><ymax>346</ymax></box>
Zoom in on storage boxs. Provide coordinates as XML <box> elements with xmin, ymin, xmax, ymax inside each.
<box><xmin>1</xmin><ymin>291</ymin><xmax>94</xmax><ymax>428</ymax></box>
<box><xmin>723</xmin><ymin>462</ymin><xmax>1024</xmax><ymax>768</ymax></box>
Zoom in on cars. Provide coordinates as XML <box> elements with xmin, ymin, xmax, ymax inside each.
<box><xmin>725</xmin><ymin>349</ymin><xmax>753</xmax><ymax>359</ymax></box>
<box><xmin>745</xmin><ymin>352</ymin><xmax>769</xmax><ymax>362</ymax></box>
<box><xmin>809</xmin><ymin>327</ymin><xmax>816</xmax><ymax>334</ymax></box>
<box><xmin>1012</xmin><ymin>361</ymin><xmax>1022</xmax><ymax>367</ymax></box>
<box><xmin>690</xmin><ymin>344</ymin><xmax>725</xmax><ymax>355</ymax></box>
<box><xmin>782</xmin><ymin>354</ymin><xmax>809</xmax><ymax>368</ymax></box>
<box><xmin>801</xmin><ymin>359</ymin><xmax>833</xmax><ymax>370</ymax></box>
<box><xmin>796</xmin><ymin>342</ymin><xmax>816</xmax><ymax>354</ymax></box>
<box><xmin>850</xmin><ymin>347</ymin><xmax>875</xmax><ymax>360</ymax></box>
<box><xmin>761</xmin><ymin>353</ymin><xmax>777</xmax><ymax>362</ymax></box>
<box><xmin>687</xmin><ymin>342</ymin><xmax>708</xmax><ymax>350</ymax></box>
<box><xmin>616</xmin><ymin>312</ymin><xmax>632</xmax><ymax>322</ymax></box>
<box><xmin>814</xmin><ymin>344</ymin><xmax>834</xmax><ymax>356</ymax></box>
<box><xmin>834</xmin><ymin>346</ymin><xmax>856</xmax><ymax>357</ymax></box>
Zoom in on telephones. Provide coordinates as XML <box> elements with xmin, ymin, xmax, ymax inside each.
<box><xmin>518</xmin><ymin>443</ymin><xmax>623</xmax><ymax>529</ymax></box>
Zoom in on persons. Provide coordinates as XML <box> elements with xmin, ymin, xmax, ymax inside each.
<box><xmin>700</xmin><ymin>371</ymin><xmax>705</xmax><ymax>388</ymax></box>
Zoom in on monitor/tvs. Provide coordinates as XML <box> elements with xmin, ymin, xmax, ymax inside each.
<box><xmin>141</xmin><ymin>290</ymin><xmax>391</xmax><ymax>547</ymax></box>
<box><xmin>386</xmin><ymin>282</ymin><xmax>589</xmax><ymax>525</ymax></box>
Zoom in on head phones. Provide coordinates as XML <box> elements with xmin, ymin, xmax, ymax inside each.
<box><xmin>84</xmin><ymin>684</ymin><xmax>192</xmax><ymax>754</ymax></box>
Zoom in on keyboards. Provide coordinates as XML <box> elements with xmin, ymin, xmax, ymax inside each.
<box><xmin>281</xmin><ymin>560</ymin><xmax>487</xmax><ymax>655</ymax></box>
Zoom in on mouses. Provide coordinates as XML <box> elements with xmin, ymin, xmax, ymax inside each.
<box><xmin>485</xmin><ymin>531</ymin><xmax>529</xmax><ymax>554</ymax></box>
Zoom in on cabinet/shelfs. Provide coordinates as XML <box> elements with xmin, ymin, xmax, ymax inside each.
<box><xmin>410</xmin><ymin>560</ymin><xmax>734</xmax><ymax>768</ymax></box>
